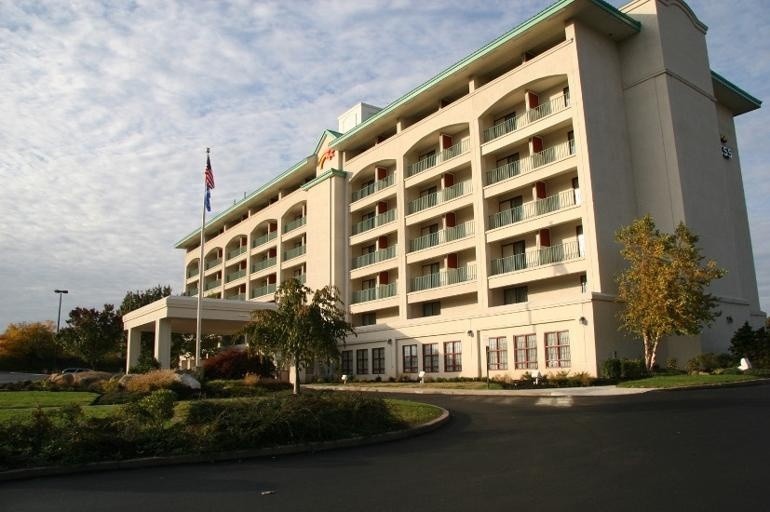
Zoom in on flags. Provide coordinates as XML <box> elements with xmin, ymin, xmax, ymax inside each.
<box><xmin>203</xmin><ymin>158</ymin><xmax>215</xmax><ymax>212</ymax></box>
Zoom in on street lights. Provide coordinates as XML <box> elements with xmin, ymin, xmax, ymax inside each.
<box><xmin>54</xmin><ymin>290</ymin><xmax>68</xmax><ymax>334</ymax></box>
